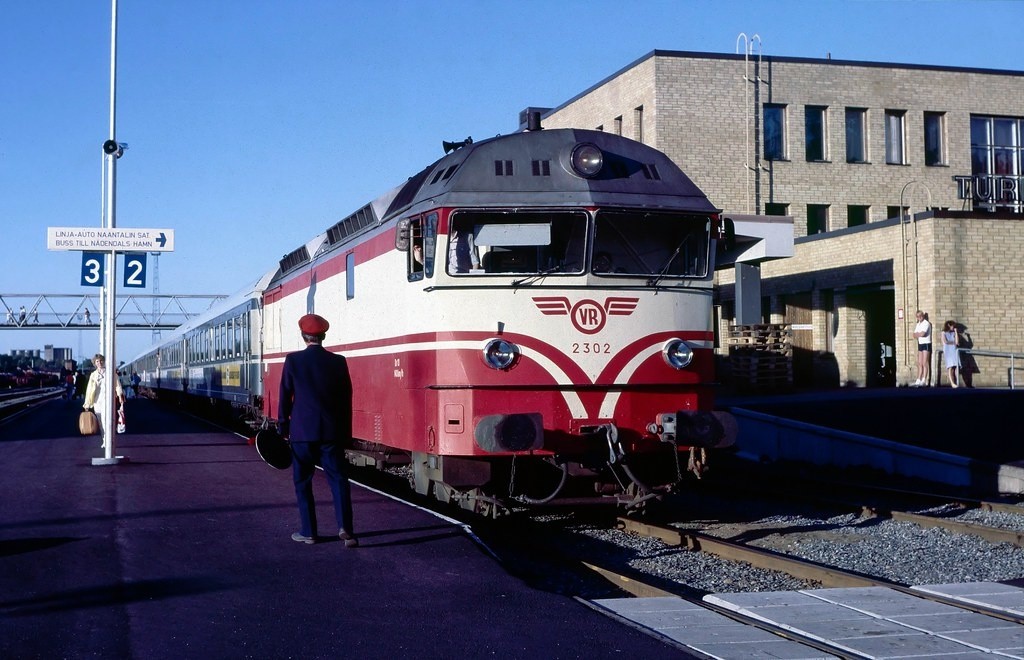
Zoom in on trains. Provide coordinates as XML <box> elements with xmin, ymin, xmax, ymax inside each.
<box><xmin>118</xmin><ymin>112</ymin><xmax>739</xmax><ymax>541</ymax></box>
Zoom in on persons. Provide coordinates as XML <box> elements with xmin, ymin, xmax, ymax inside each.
<box><xmin>276</xmin><ymin>314</ymin><xmax>361</xmax><ymax>550</ymax></box>
<box><xmin>82</xmin><ymin>353</ymin><xmax>126</xmax><ymax>449</ymax></box>
<box><xmin>591</xmin><ymin>250</ymin><xmax>617</xmax><ymax>274</ymax></box>
<box><xmin>908</xmin><ymin>310</ymin><xmax>933</xmax><ymax>388</ymax></box>
<box><xmin>412</xmin><ymin>216</ymin><xmax>481</xmax><ymax>275</ymax></box>
<box><xmin>940</xmin><ymin>320</ymin><xmax>961</xmax><ymax>389</ymax></box>
<box><xmin>62</xmin><ymin>361</ymin><xmax>142</xmax><ymax>403</ymax></box>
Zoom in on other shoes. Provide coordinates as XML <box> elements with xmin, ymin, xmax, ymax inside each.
<box><xmin>338</xmin><ymin>528</ymin><xmax>360</xmax><ymax>548</ymax></box>
<box><xmin>912</xmin><ymin>379</ymin><xmax>926</xmax><ymax>386</ymax></box>
<box><xmin>951</xmin><ymin>382</ymin><xmax>959</xmax><ymax>388</ymax></box>
<box><xmin>291</xmin><ymin>533</ymin><xmax>316</xmax><ymax>544</ymax></box>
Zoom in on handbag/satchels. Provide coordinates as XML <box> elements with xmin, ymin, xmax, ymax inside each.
<box><xmin>117</xmin><ymin>401</ymin><xmax>126</xmax><ymax>433</ymax></box>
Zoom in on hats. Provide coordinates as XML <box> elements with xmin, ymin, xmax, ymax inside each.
<box><xmin>298</xmin><ymin>314</ymin><xmax>329</xmax><ymax>336</ymax></box>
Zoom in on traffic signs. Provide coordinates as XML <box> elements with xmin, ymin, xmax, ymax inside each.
<box><xmin>46</xmin><ymin>224</ymin><xmax>174</xmax><ymax>253</ymax></box>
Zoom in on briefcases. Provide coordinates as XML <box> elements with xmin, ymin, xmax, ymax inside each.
<box><xmin>79</xmin><ymin>409</ymin><xmax>99</xmax><ymax>435</ymax></box>
